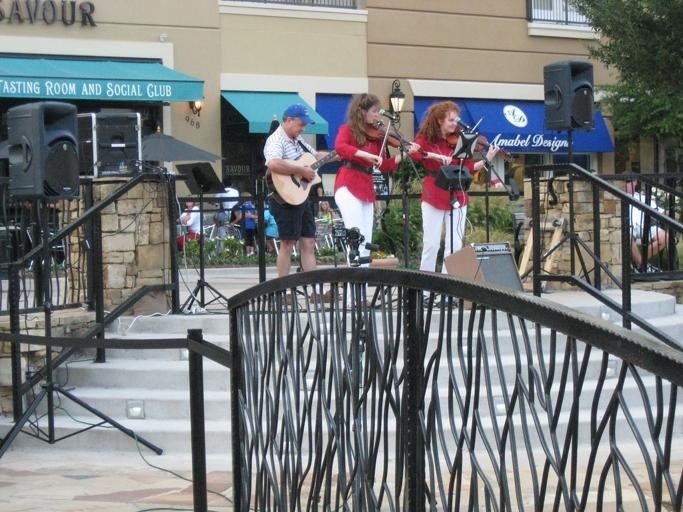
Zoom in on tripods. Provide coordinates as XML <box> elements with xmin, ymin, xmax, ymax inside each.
<box><xmin>518</xmin><ymin>132</ymin><xmax>622</xmax><ymax>292</ymax></box>
<box><xmin>175</xmin><ymin>203</ymin><xmax>230</xmax><ymax>314</ymax></box>
<box><xmin>0</xmin><ymin>204</ymin><xmax>179</xmax><ymax>463</ymax></box>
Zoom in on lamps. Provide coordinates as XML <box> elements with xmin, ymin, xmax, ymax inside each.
<box><xmin>188</xmin><ymin>93</ymin><xmax>205</xmax><ymax>119</ymax></box>
<box><xmin>386</xmin><ymin>80</ymin><xmax>406</xmax><ymax>125</ymax></box>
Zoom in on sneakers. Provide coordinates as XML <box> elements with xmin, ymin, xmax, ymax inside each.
<box><xmin>309</xmin><ymin>290</ymin><xmax>343</xmax><ymax>303</ymax></box>
<box><xmin>630</xmin><ymin>260</ymin><xmax>665</xmax><ymax>275</ymax></box>
<box><xmin>281</xmin><ymin>295</ymin><xmax>294</xmax><ymax>305</ymax></box>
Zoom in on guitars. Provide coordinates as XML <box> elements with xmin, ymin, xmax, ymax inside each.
<box><xmin>266</xmin><ymin>150</ymin><xmax>339</xmax><ymax>209</ymax></box>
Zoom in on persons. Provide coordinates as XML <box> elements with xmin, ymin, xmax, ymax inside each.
<box><xmin>622</xmin><ymin>170</ymin><xmax>680</xmax><ymax>274</ymax></box>
<box><xmin>262</xmin><ymin>103</ymin><xmax>343</xmax><ymax>305</ymax></box>
<box><xmin>407</xmin><ymin>101</ymin><xmax>500</xmax><ymax>305</ymax></box>
<box><xmin>334</xmin><ymin>93</ymin><xmax>421</xmax><ymax>307</ymax></box>
<box><xmin>176</xmin><ymin>178</ymin><xmax>337</xmax><ymax>257</ymax></box>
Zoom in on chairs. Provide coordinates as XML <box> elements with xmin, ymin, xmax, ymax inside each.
<box><xmin>169</xmin><ymin>212</ymin><xmax>346</xmax><ymax>257</ymax></box>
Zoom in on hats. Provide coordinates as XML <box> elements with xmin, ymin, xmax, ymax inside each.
<box><xmin>283</xmin><ymin>103</ymin><xmax>316</xmax><ymax>125</ymax></box>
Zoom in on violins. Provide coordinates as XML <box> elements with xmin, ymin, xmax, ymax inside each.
<box><xmin>359</xmin><ymin>119</ymin><xmax>429</xmax><ymax>158</ymax></box>
<box><xmin>447</xmin><ymin>129</ymin><xmax>512</xmax><ymax>158</ymax></box>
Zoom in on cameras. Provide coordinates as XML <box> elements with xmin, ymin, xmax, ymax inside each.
<box><xmin>332</xmin><ymin>226</ymin><xmax>365</xmax><ymax>248</ymax></box>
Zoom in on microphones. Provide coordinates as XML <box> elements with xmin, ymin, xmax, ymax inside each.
<box><xmin>456</xmin><ymin>118</ymin><xmax>473</xmax><ymax>130</ymax></box>
<box><xmin>377</xmin><ymin>107</ymin><xmax>400</xmax><ymax>125</ymax></box>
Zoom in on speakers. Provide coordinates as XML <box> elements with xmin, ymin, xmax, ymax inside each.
<box><xmin>79</xmin><ymin>112</ymin><xmax>144</xmax><ymax>176</ymax></box>
<box><xmin>6</xmin><ymin>101</ymin><xmax>82</xmax><ymax>202</ymax></box>
<box><xmin>441</xmin><ymin>240</ymin><xmax>524</xmax><ymax>306</ymax></box>
<box><xmin>543</xmin><ymin>61</ymin><xmax>595</xmax><ymax>131</ymax></box>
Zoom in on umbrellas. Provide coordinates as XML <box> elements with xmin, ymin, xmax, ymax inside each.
<box><xmin>142</xmin><ymin>125</ymin><xmax>227</xmax><ymax>163</ymax></box>
